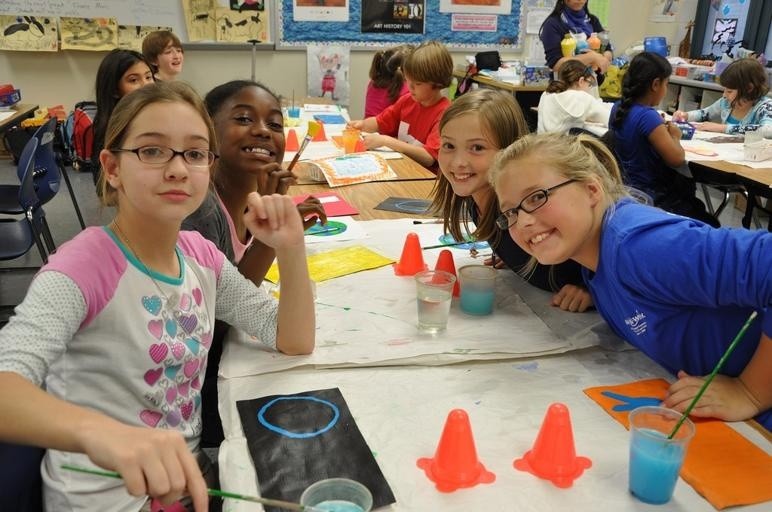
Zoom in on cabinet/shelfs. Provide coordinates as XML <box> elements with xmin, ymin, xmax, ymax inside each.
<box><xmin>655</xmin><ymin>73</ymin><xmax>725</xmax><ymax>118</ymax></box>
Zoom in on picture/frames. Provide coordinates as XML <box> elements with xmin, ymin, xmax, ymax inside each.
<box><xmin>689</xmin><ymin>0</ymin><xmax>764</xmax><ymax>60</ymax></box>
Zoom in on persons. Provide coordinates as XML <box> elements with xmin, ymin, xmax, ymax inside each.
<box><xmin>185</xmin><ymin>81</ymin><xmax>328</xmax><ymax>449</ymax></box>
<box><xmin>361</xmin><ymin>43</ymin><xmax>410</xmax><ymax>125</ymax></box>
<box><xmin>87</xmin><ymin>47</ymin><xmax>158</xmax><ymax>189</ymax></box>
<box><xmin>344</xmin><ymin>40</ymin><xmax>455</xmax><ymax>176</ymax></box>
<box><xmin>423</xmin><ymin>85</ymin><xmax>597</xmax><ymax>316</ymax></box>
<box><xmin>137</xmin><ymin>28</ymin><xmax>189</xmax><ymax>84</ymax></box>
<box><xmin>605</xmin><ymin>50</ymin><xmax>725</xmax><ymax>230</ymax></box>
<box><xmin>533</xmin><ymin>58</ymin><xmax>615</xmax><ymax>139</ymax></box>
<box><xmin>670</xmin><ymin>57</ymin><xmax>770</xmax><ymax>141</ymax></box>
<box><xmin>537</xmin><ymin>0</ymin><xmax>616</xmax><ymax>80</ymax></box>
<box><xmin>483</xmin><ymin>126</ymin><xmax>770</xmax><ymax>424</ymax></box>
<box><xmin>0</xmin><ymin>80</ymin><xmax>319</xmax><ymax>512</ymax></box>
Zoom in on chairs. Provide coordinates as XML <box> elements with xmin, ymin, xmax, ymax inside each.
<box><xmin>1</xmin><ymin>137</ymin><xmax>56</xmax><ymax>271</ymax></box>
<box><xmin>570</xmin><ymin>127</ymin><xmax>626</xmax><ymax>188</ymax></box>
<box><xmin>1</xmin><ymin>118</ymin><xmax>90</xmax><ymax>233</ymax></box>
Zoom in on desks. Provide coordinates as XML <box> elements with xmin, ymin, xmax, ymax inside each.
<box><xmin>531</xmin><ymin>94</ymin><xmax>772</xmax><ymax>203</ymax></box>
<box><xmin>1</xmin><ymin>102</ymin><xmax>40</xmax><ymax>130</ymax></box>
<box><xmin>735</xmin><ymin>169</ymin><xmax>772</xmax><ymax>200</ymax></box>
<box><xmin>224</xmin><ymin>178</ymin><xmax>772</xmax><ymax>511</ymax></box>
<box><xmin>278</xmin><ymin>94</ymin><xmax>439</xmax><ymax>185</ymax></box>
<box><xmin>450</xmin><ymin>69</ymin><xmax>553</xmax><ymax>96</ymax></box>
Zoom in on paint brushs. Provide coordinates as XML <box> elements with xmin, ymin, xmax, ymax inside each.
<box><xmin>305</xmin><ymin>227</ymin><xmax>341</xmax><ymax>235</ymax></box>
<box><xmin>414</xmin><ymin>219</ymin><xmax>474</xmax><ymax>225</ymax></box>
<box><xmin>344</xmin><ymin>120</ymin><xmax>366</xmax><ymax>139</ymax></box>
<box><xmin>423</xmin><ymin>240</ymin><xmax>477</xmax><ymax>250</ymax></box>
<box><xmin>60</xmin><ymin>464</ymin><xmax>327</xmax><ymax>512</ymax></box>
<box><xmin>680</xmin><ymin>111</ymin><xmax>684</xmax><ymax>123</ymax></box>
<box><xmin>291</xmin><ymin>89</ymin><xmax>294</xmax><ymax>108</ymax></box>
<box><xmin>286</xmin><ymin>122</ymin><xmax>321</xmax><ymax>171</ymax></box>
<box><xmin>661</xmin><ymin>311</ymin><xmax>759</xmax><ymax>449</ymax></box>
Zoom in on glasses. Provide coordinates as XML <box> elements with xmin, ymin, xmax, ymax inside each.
<box><xmin>104</xmin><ymin>140</ymin><xmax>225</xmax><ymax>170</ymax></box>
<box><xmin>491</xmin><ymin>174</ymin><xmax>583</xmax><ymax>233</ymax></box>
<box><xmin>584</xmin><ymin>78</ymin><xmax>595</xmax><ymax>87</ymax></box>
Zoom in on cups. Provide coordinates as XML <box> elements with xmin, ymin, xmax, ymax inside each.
<box><xmin>301</xmin><ymin>479</ymin><xmax>373</xmax><ymax>512</ymax></box>
<box><xmin>415</xmin><ymin>271</ymin><xmax>456</xmax><ymax>333</ymax></box>
<box><xmin>288</xmin><ymin>100</ymin><xmax>300</xmax><ymax>119</ymax></box>
<box><xmin>459</xmin><ymin>264</ymin><xmax>497</xmax><ymax>315</ymax></box>
<box><xmin>628</xmin><ymin>406</ymin><xmax>695</xmax><ymax>506</ymax></box>
